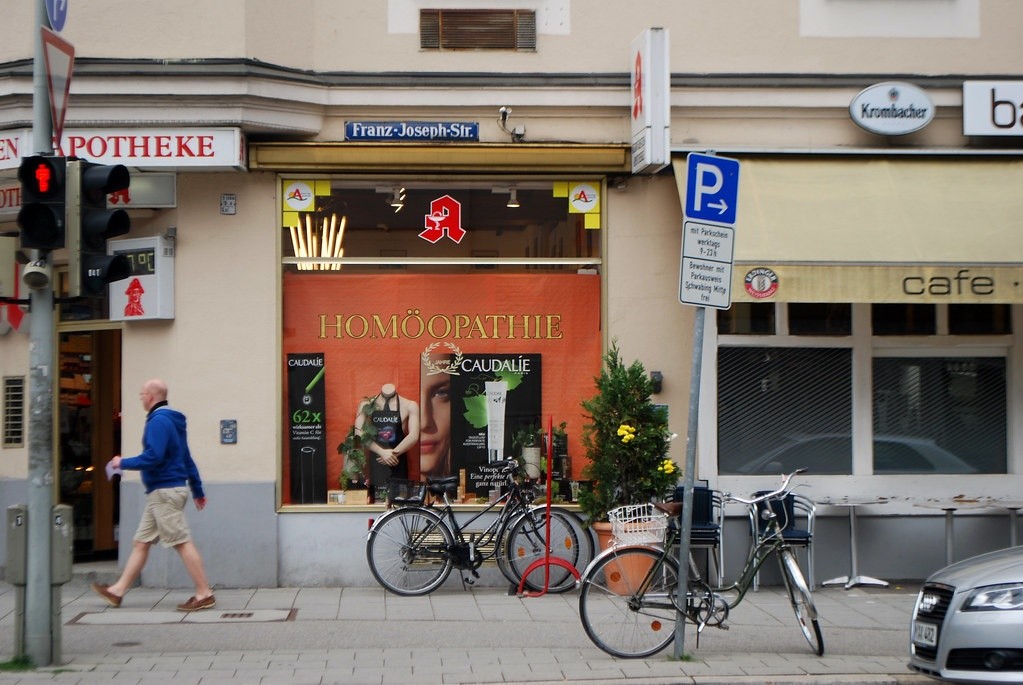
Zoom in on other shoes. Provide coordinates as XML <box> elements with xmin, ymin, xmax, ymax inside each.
<box><xmin>90</xmin><ymin>582</ymin><xmax>122</xmax><ymax>608</ymax></box>
<box><xmin>176</xmin><ymin>595</ymin><xmax>217</xmax><ymax>611</ymax></box>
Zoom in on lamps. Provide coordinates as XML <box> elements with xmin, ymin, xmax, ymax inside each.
<box><xmin>507</xmin><ymin>188</ymin><xmax>520</xmax><ymax>208</ymax></box>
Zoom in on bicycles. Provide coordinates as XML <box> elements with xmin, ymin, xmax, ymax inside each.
<box><xmin>578</xmin><ymin>462</ymin><xmax>825</xmax><ymax>663</ymax></box>
<box><xmin>364</xmin><ymin>456</ymin><xmax>597</xmax><ymax>597</ymax></box>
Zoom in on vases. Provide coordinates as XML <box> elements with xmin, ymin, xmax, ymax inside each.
<box><xmin>592</xmin><ymin>521</ymin><xmax>657</xmax><ymax>595</ymax></box>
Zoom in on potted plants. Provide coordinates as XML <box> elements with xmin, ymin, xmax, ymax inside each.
<box><xmin>511</xmin><ymin>424</ymin><xmax>544</xmax><ymax>479</ymax></box>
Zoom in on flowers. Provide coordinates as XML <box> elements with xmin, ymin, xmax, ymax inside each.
<box><xmin>576</xmin><ymin>335</ymin><xmax>683</xmax><ymax>529</ymax></box>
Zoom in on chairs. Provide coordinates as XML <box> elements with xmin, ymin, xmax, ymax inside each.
<box><xmin>662</xmin><ymin>486</ymin><xmax>817</xmax><ymax>593</ymax></box>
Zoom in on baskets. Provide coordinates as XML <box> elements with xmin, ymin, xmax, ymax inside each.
<box><xmin>606</xmin><ymin>502</ymin><xmax>669</xmax><ymax>546</ymax></box>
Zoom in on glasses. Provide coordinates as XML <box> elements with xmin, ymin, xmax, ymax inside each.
<box><xmin>139</xmin><ymin>391</ymin><xmax>149</xmax><ymax>397</ymax></box>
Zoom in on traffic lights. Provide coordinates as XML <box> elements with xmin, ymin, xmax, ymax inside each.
<box><xmin>14</xmin><ymin>155</ymin><xmax>67</xmax><ymax>254</ymax></box>
<box><xmin>72</xmin><ymin>154</ymin><xmax>132</xmax><ymax>319</ymax></box>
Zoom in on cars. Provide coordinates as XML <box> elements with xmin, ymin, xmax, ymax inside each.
<box><xmin>905</xmin><ymin>544</ymin><xmax>1022</xmax><ymax>685</ymax></box>
<box><xmin>734</xmin><ymin>434</ymin><xmax>983</xmax><ymax>476</ymax></box>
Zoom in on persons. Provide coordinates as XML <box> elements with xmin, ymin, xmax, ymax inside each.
<box><xmin>354</xmin><ymin>383</ymin><xmax>420</xmax><ymax>504</ymax></box>
<box><xmin>419</xmin><ymin>353</ymin><xmax>451</xmax><ymax>504</ymax></box>
<box><xmin>91</xmin><ymin>378</ymin><xmax>217</xmax><ymax>612</ymax></box>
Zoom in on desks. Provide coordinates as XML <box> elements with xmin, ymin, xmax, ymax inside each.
<box><xmin>913</xmin><ymin>494</ymin><xmax>998</xmax><ymax>568</ymax></box>
<box><xmin>988</xmin><ymin>495</ymin><xmax>1023</xmax><ymax>547</ymax></box>
<box><xmin>816</xmin><ymin>496</ymin><xmax>890</xmax><ymax>590</ymax></box>
